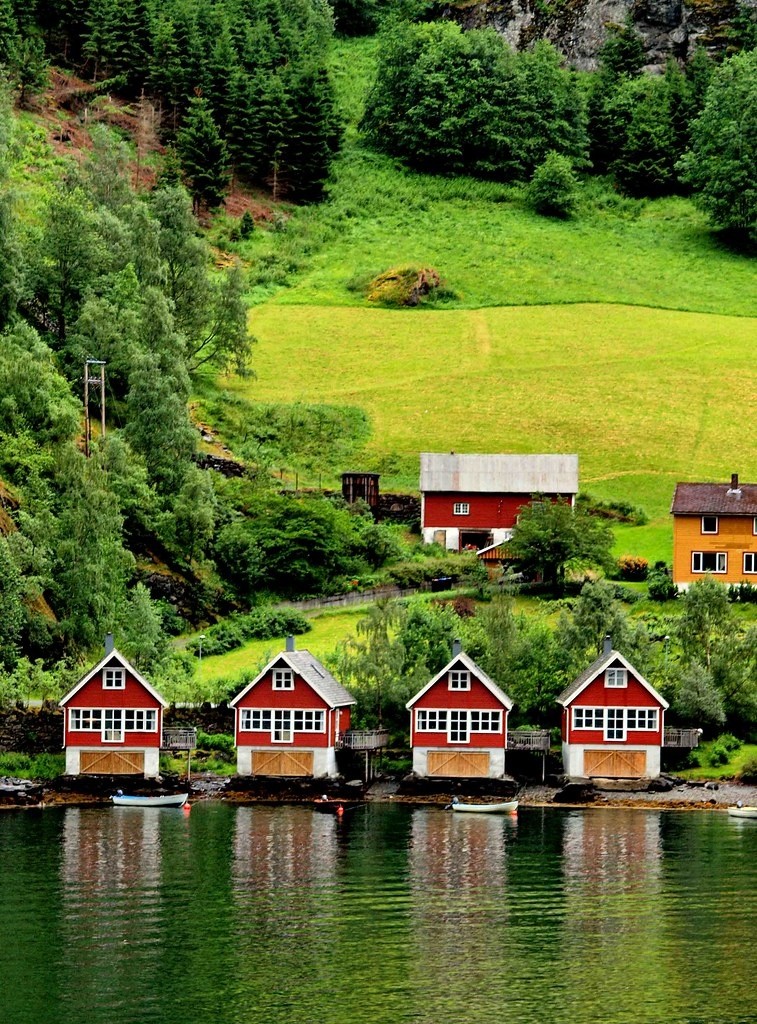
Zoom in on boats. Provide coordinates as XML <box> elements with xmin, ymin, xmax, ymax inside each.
<box><xmin>109</xmin><ymin>793</ymin><xmax>188</xmax><ymax>808</ymax></box>
